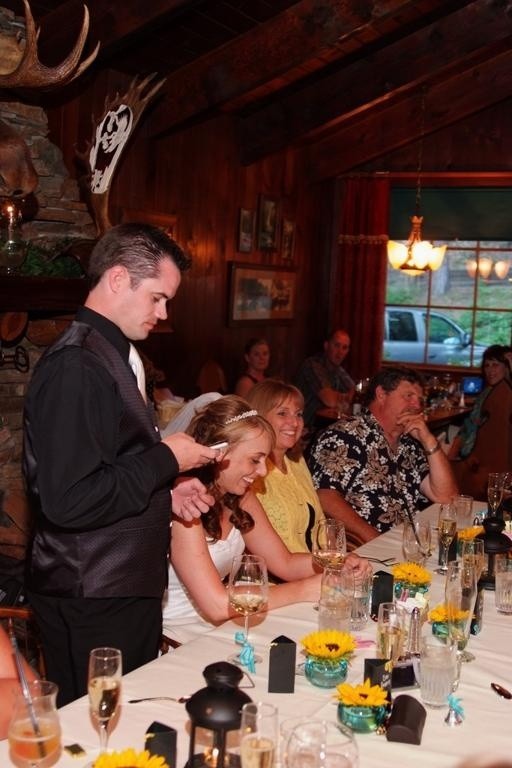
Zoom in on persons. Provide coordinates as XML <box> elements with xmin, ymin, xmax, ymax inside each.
<box><xmin>1</xmin><ymin>610</ymin><xmax>45</xmax><ymax>739</ymax></box>
<box><xmin>24</xmin><ymin>225</ymin><xmax>223</xmax><ymax>711</ymax></box>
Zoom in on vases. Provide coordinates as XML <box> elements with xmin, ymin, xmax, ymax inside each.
<box><xmin>305</xmin><ymin>658</ymin><xmax>347</xmax><ymax>686</ymax></box>
<box><xmin>459</xmin><ymin>542</ymin><xmax>480</xmax><ymax>555</ymax></box>
<box><xmin>395</xmin><ymin>585</ymin><xmax>430</xmax><ymax>600</ymax></box>
<box><xmin>337</xmin><ymin>701</ymin><xmax>387</xmax><ymax>733</ymax></box>
<box><xmin>433</xmin><ymin>620</ymin><xmax>467</xmax><ymax>649</ymax></box>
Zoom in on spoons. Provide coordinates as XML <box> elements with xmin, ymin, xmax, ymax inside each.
<box><xmin>128</xmin><ymin>696</ymin><xmax>192</xmax><ymax>706</ymax></box>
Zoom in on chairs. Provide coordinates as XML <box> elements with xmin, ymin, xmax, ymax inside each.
<box><xmin>448</xmin><ymin>377</ymin><xmax>512</xmax><ymax>501</ymax></box>
<box><xmin>0</xmin><ymin>604</ymin><xmax>50</xmax><ymax>690</ymax></box>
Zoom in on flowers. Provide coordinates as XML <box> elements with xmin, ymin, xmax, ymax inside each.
<box><xmin>93</xmin><ymin>746</ymin><xmax>171</xmax><ymax>768</ymax></box>
<box><xmin>455</xmin><ymin>524</ymin><xmax>486</xmax><ymax>539</ymax></box>
<box><xmin>298</xmin><ymin>627</ymin><xmax>358</xmax><ymax>660</ymax></box>
<box><xmin>330</xmin><ymin>677</ymin><xmax>389</xmax><ymax>710</ymax></box>
<box><xmin>425</xmin><ymin>602</ymin><xmax>477</xmax><ymax>628</ymax></box>
<box><xmin>391</xmin><ymin>561</ymin><xmax>431</xmax><ymax>585</ymax></box>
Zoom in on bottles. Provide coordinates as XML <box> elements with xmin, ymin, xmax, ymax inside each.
<box><xmin>329</xmin><ymin>370</ymin><xmax>468</xmax><ymax>425</ymax></box>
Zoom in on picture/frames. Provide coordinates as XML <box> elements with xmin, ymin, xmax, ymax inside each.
<box><xmin>278</xmin><ymin>219</ymin><xmax>297</xmax><ymax>261</ymax></box>
<box><xmin>254</xmin><ymin>192</ymin><xmax>281</xmax><ymax>252</ymax></box>
<box><xmin>236</xmin><ymin>205</ymin><xmax>255</xmax><ymax>254</ymax></box>
<box><xmin>223</xmin><ymin>259</ymin><xmax>300</xmax><ymax>327</ymax></box>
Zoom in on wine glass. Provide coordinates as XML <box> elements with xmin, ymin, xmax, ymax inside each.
<box><xmin>230</xmin><ymin>553</ymin><xmax>269</xmax><ymax>669</ymax></box>
<box><xmin>448</xmin><ymin>492</ymin><xmax>473</xmax><ymax>528</ymax></box>
<box><xmin>87</xmin><ymin>646</ymin><xmax>124</xmax><ymax>757</ymax></box>
<box><xmin>458</xmin><ymin>537</ymin><xmax>484</xmax><ymax>586</ymax></box>
<box><xmin>7</xmin><ymin>680</ymin><xmax>63</xmax><ymax>767</ymax></box>
<box><xmin>485</xmin><ymin>471</ymin><xmax>508</xmax><ymax>516</ymax></box>
<box><xmin>402</xmin><ymin>515</ymin><xmax>431</xmax><ymax>568</ymax></box>
<box><xmin>437</xmin><ymin>502</ymin><xmax>458</xmax><ymax>575</ymax></box>
<box><xmin>318</xmin><ymin>562</ymin><xmax>355</xmax><ymax>642</ymax></box>
<box><xmin>378</xmin><ymin>602</ymin><xmax>405</xmax><ymax>664</ymax></box>
<box><xmin>444</xmin><ymin>559</ymin><xmax>476</xmax><ymax>662</ymax></box>
<box><xmin>312</xmin><ymin>517</ymin><xmax>347</xmax><ymax>614</ymax></box>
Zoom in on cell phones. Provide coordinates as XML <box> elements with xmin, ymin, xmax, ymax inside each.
<box><xmin>206</xmin><ymin>439</ymin><xmax>229</xmax><ymax>449</ymax></box>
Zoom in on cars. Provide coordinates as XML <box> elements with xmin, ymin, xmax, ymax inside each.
<box><xmin>383</xmin><ymin>304</ymin><xmax>495</xmax><ymax>368</ymax></box>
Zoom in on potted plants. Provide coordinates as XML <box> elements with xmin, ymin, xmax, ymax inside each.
<box><xmin>0</xmin><ymin>238</ymin><xmax>89</xmax><ymax>314</ymax></box>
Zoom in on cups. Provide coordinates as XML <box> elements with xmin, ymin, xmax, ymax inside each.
<box><xmin>236</xmin><ymin>699</ymin><xmax>360</xmax><ymax>768</ymax></box>
<box><xmin>344</xmin><ymin>568</ymin><xmax>369</xmax><ymax>631</ymax></box>
<box><xmin>493</xmin><ymin>552</ymin><xmax>512</xmax><ymax>616</ymax></box>
<box><xmin>412</xmin><ymin>636</ymin><xmax>462</xmax><ymax>709</ymax></box>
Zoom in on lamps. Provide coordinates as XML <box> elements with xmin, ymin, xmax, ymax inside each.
<box><xmin>384</xmin><ymin>80</ymin><xmax>448</xmax><ymax>279</ymax></box>
<box><xmin>472</xmin><ymin>514</ymin><xmax>512</xmax><ymax>590</ymax></box>
<box><xmin>182</xmin><ymin>662</ymin><xmax>257</xmax><ymax>768</ymax></box>
<box><xmin>0</xmin><ymin>200</ymin><xmax>30</xmax><ymax>275</ymax></box>
